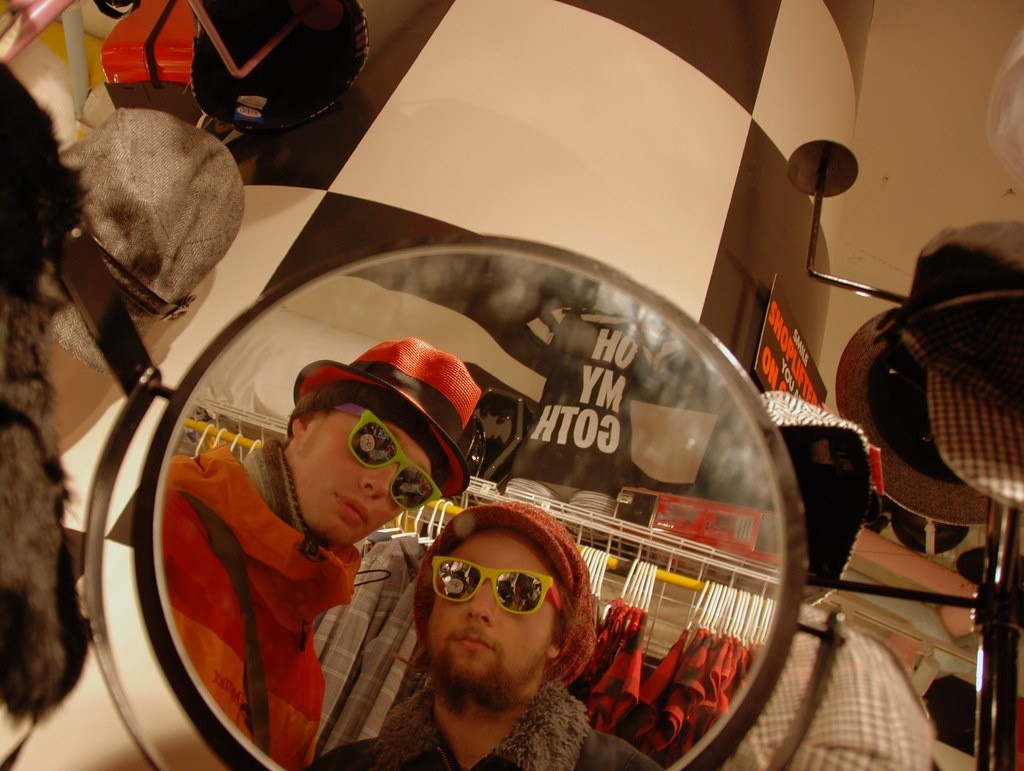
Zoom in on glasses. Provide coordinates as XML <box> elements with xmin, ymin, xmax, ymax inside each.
<box><xmin>430</xmin><ymin>555</ymin><xmax>562</xmax><ymax>614</ymax></box>
<box><xmin>335</xmin><ymin>403</ymin><xmax>443</xmax><ymax>510</ymax></box>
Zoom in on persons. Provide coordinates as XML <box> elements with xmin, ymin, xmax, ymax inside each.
<box><xmin>300</xmin><ymin>503</ymin><xmax>663</xmax><ymax>771</ymax></box>
<box><xmin>157</xmin><ymin>336</ymin><xmax>480</xmax><ymax>771</ymax></box>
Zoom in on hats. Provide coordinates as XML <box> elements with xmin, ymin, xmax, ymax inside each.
<box><xmin>900</xmin><ymin>222</ymin><xmax>1024</xmax><ymax>509</ymax></box>
<box><xmin>724</xmin><ymin>616</ymin><xmax>935</xmax><ymax>771</ymax></box>
<box><xmin>891</xmin><ymin>508</ymin><xmax>972</xmax><ymax>554</ymax></box>
<box><xmin>190</xmin><ymin>0</ymin><xmax>369</xmax><ymax>130</ymax></box>
<box><xmin>835</xmin><ymin>306</ymin><xmax>992</xmax><ymax>527</ymax></box>
<box><xmin>292</xmin><ymin>338</ymin><xmax>482</xmax><ymax>497</ymax></box>
<box><xmin>37</xmin><ymin>106</ymin><xmax>245</xmax><ymax>376</ymax></box>
<box><xmin>757</xmin><ymin>390</ymin><xmax>874</xmax><ymax>609</ymax></box>
<box><xmin>412</xmin><ymin>501</ymin><xmax>596</xmax><ymax>684</ymax></box>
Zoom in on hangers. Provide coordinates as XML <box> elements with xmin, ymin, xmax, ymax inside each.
<box><xmin>189</xmin><ymin>425</ymin><xmax>776</xmax><ymax>655</ymax></box>
